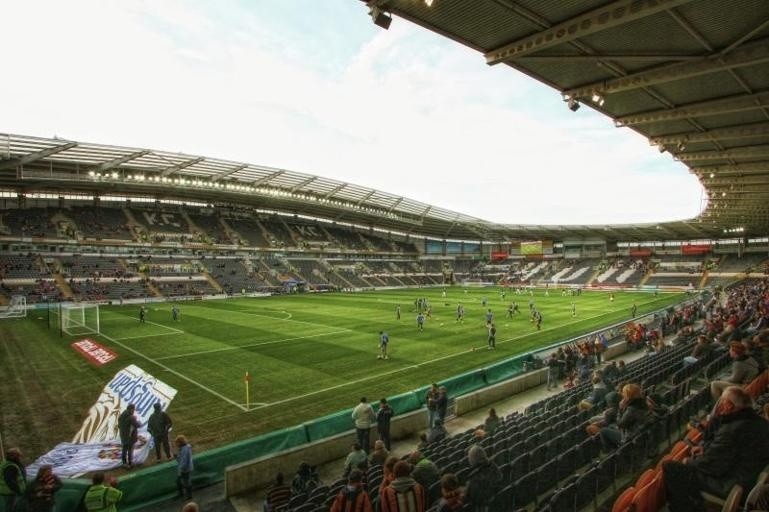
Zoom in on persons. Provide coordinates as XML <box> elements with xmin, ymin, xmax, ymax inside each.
<box><xmin>0</xmin><ymin>382</ymin><xmax>510</xmax><ymax>512</ymax></box>
<box><xmin>375</xmin><ymin>286</ymin><xmax>580</xmax><ymax>360</ymax></box>
<box><xmin>466</xmin><ymin>255</ymin><xmax>768</xmax><ymax>292</ymax></box>
<box><xmin>2</xmin><ymin>207</ymin><xmax>445</xmax><ymax>322</ymax></box>
<box><xmin>544</xmin><ymin>280</ymin><xmax>769</xmax><ymax>512</ymax></box>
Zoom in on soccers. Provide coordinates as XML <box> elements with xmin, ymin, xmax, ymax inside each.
<box><xmin>440</xmin><ymin>322</ymin><xmax>443</xmax><ymax>327</ymax></box>
<box><xmin>435</xmin><ymin>318</ymin><xmax>440</xmax><ymax>321</ymax></box>
<box><xmin>530</xmin><ymin>319</ymin><xmax>534</xmax><ymax>323</ymax></box>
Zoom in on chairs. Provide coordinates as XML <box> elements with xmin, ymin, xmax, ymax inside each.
<box><xmin>277</xmin><ymin>327</ymin><xmax>769</xmax><ymax>511</ymax></box>
